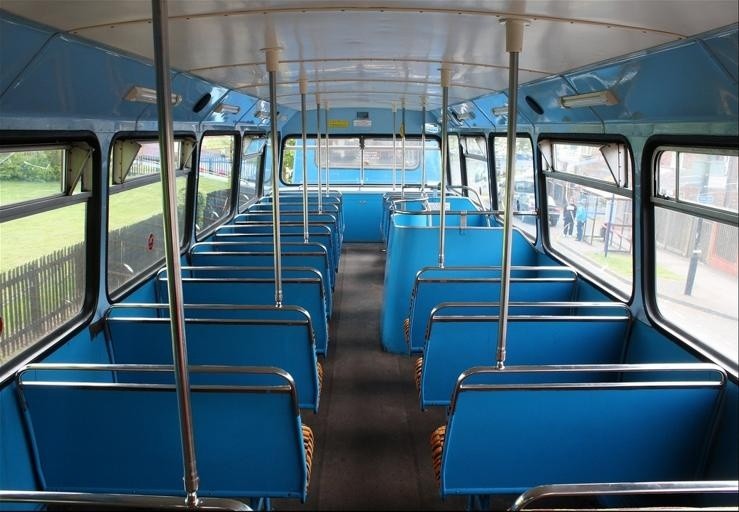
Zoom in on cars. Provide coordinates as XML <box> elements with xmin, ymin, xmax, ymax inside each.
<box><xmin>474</xmin><ymin>167</ymin><xmax>560</xmax><ymax>226</ymax></box>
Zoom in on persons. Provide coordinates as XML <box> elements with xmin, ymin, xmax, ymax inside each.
<box><xmin>574</xmin><ymin>201</ymin><xmax>588</xmax><ymax>241</ymax></box>
<box><xmin>563</xmin><ymin>198</ymin><xmax>578</xmax><ymax>237</ymax></box>
